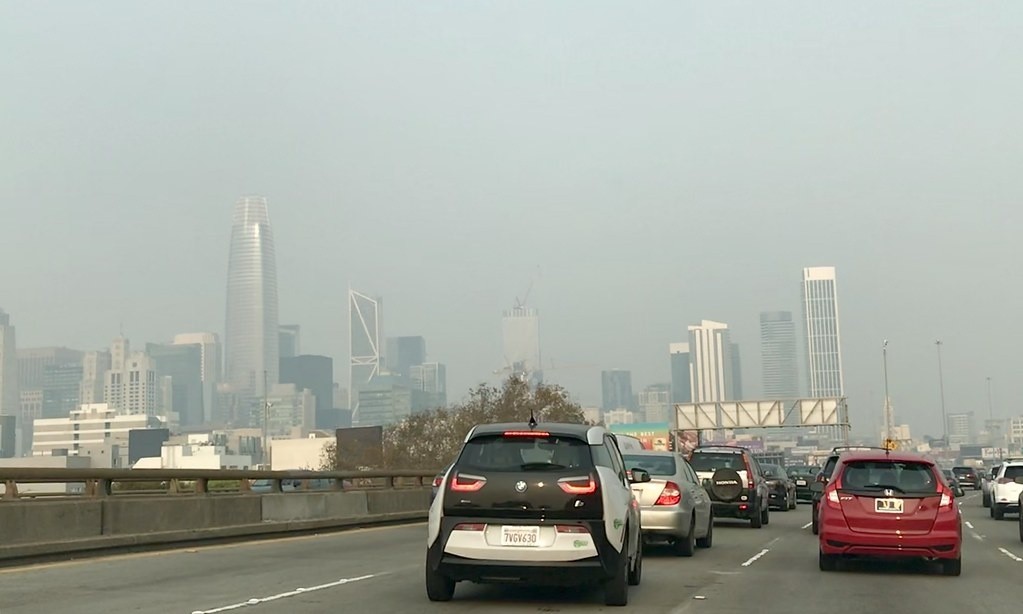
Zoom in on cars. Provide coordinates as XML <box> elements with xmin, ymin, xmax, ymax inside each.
<box><xmin>617</xmin><ymin>442</ymin><xmax>1022</xmax><ymax>576</ymax></box>
<box><xmin>426</xmin><ymin>408</ymin><xmax>643</xmax><ymax>607</ymax></box>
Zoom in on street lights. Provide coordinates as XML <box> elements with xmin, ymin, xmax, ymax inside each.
<box><xmin>881</xmin><ymin>337</ymin><xmax>950</xmax><ymax>460</ymax></box>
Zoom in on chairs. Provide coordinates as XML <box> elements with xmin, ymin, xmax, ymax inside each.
<box><xmin>849</xmin><ymin>466</ymin><xmax>870</xmax><ymax>487</ymax></box>
<box><xmin>480</xmin><ymin>441</ymin><xmax>525</xmax><ymax>466</ymax></box>
<box><xmin>551</xmin><ymin>447</ymin><xmax>581</xmax><ymax>468</ymax></box>
